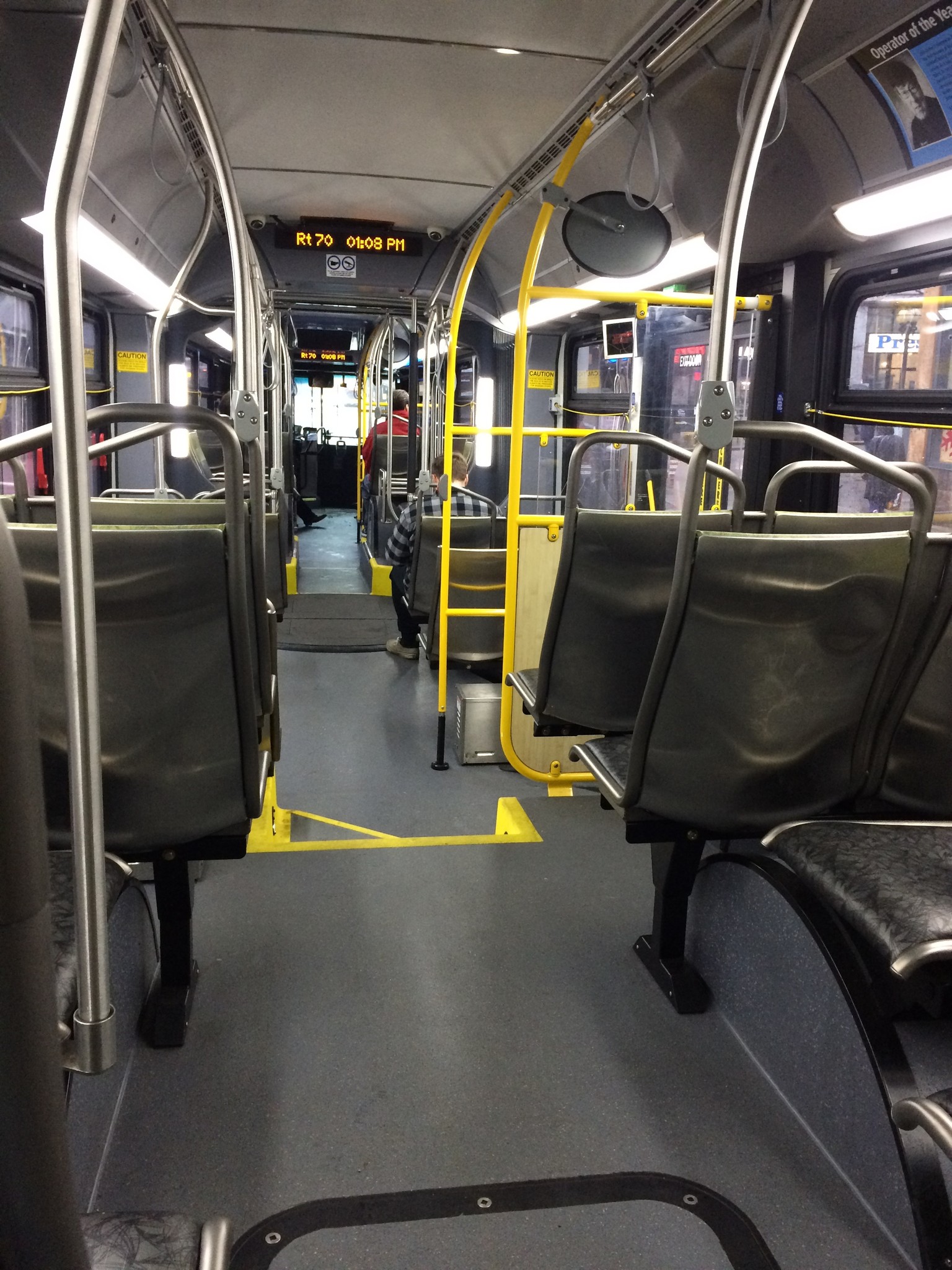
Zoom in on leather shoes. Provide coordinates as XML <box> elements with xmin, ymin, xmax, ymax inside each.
<box><xmin>304</xmin><ymin>514</ymin><xmax>327</xmax><ymax>527</ymax></box>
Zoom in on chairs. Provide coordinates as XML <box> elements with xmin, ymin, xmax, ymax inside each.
<box><xmin>0</xmin><ymin>496</ymin><xmax>235</xmax><ymax>1269</ymax></box>
<box><xmin>504</xmin><ymin>432</ymin><xmax>747</xmax><ymax>738</ymax></box>
<box><xmin>47</xmin><ymin>847</ymin><xmax>132</xmax><ymax>1056</ymax></box>
<box><xmin>759</xmin><ymin>821</ymin><xmax>952</xmax><ymax>981</ymax></box>
<box><xmin>0</xmin><ymin>401</ymin><xmax>273</xmax><ymax>1052</ymax></box>
<box><xmin>568</xmin><ymin>419</ymin><xmax>934</xmax><ymax>1016</ymax></box>
<box><xmin>760</xmin><ymin>459</ymin><xmax>938</xmax><ymax>535</ymax></box>
<box><xmin>1</xmin><ymin>413</ymin><xmax>517</xmax><ymax>764</ymax></box>
<box><xmin>857</xmin><ymin>539</ymin><xmax>952</xmax><ymax>821</ymax></box>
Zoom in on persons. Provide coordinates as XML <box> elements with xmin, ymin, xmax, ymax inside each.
<box><xmin>359</xmin><ymin>389</ymin><xmax>420</xmax><ymax>538</ymax></box>
<box><xmin>219</xmin><ymin>391</ymin><xmax>231</xmax><ymax>416</ymax></box>
<box><xmin>384</xmin><ymin>451</ymin><xmax>505</xmax><ymax>659</ymax></box>
<box><xmin>864</xmin><ymin>426</ymin><xmax>906</xmax><ymax>513</ymax></box>
<box><xmin>296</xmin><ymin>492</ymin><xmax>327</xmax><ymax>527</ymax></box>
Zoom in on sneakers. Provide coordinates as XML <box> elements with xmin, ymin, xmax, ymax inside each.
<box><xmin>386</xmin><ymin>637</ymin><xmax>419</xmax><ymax>659</ymax></box>
